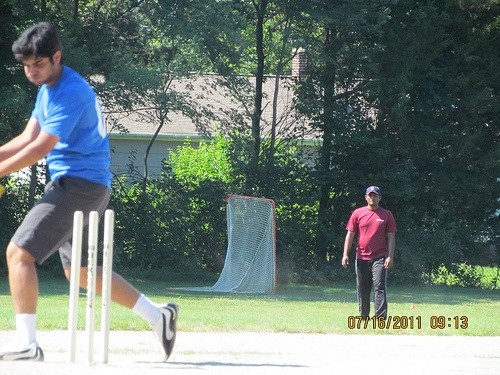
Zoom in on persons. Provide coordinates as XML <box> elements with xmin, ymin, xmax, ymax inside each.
<box><xmin>342</xmin><ymin>186</ymin><xmax>397</xmax><ymax>320</ymax></box>
<box><xmin>1</xmin><ymin>23</ymin><xmax>178</xmax><ymax>363</ymax></box>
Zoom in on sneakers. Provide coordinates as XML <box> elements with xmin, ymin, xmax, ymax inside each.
<box><xmin>147</xmin><ymin>302</ymin><xmax>178</xmax><ymax>360</ymax></box>
<box><xmin>0</xmin><ymin>342</ymin><xmax>44</xmax><ymax>361</ymax></box>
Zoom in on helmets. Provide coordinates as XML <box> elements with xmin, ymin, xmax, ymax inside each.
<box><xmin>366</xmin><ymin>186</ymin><xmax>381</xmax><ymax>195</ymax></box>
